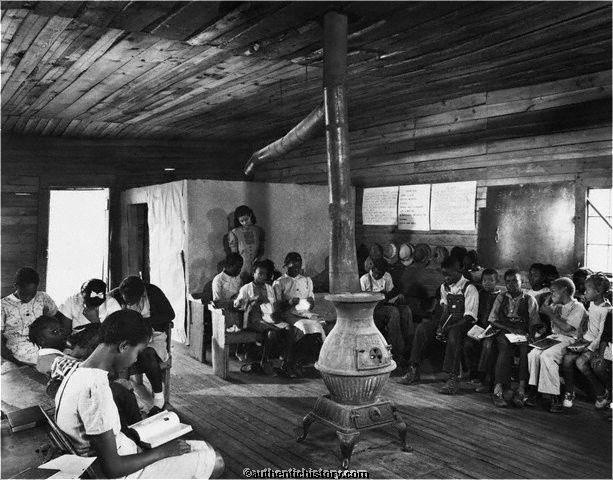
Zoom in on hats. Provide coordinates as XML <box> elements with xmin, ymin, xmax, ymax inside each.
<box><xmin>369</xmin><ymin>243</ymin><xmax>477</xmax><ymax>265</ymax></box>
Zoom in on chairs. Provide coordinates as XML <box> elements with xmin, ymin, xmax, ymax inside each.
<box><xmin>134</xmin><ymin>322</ymin><xmax>174</xmax><ymax>404</ymax></box>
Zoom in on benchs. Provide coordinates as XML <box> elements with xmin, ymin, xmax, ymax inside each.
<box><xmin>186</xmin><ymin>290</ymin><xmax>326</xmax><ymax>378</ymax></box>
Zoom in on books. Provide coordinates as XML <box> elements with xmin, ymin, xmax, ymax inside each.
<box><xmin>6</xmin><ymin>404</ymin><xmax>47</xmax><ymax>433</ymax></box>
<box><xmin>127</xmin><ymin>408</ymin><xmax>195</xmax><ymax>451</ymax></box>
<box><xmin>8</xmin><ymin>466</ymin><xmax>61</xmax><ymax>479</ymax></box>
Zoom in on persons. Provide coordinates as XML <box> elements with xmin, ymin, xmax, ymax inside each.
<box><xmin>359</xmin><ymin>240</ymin><xmax>612</xmax><ymax>412</ymax></box>
<box><xmin>208</xmin><ymin>252</ymin><xmax>327</xmax><ymax>380</ymax></box>
<box><xmin>224</xmin><ymin>204</ymin><xmax>266</xmax><ymax>274</ymax></box>
<box><xmin>1</xmin><ymin>266</ymin><xmax>176</xmax><ymax>425</ymax></box>
<box><xmin>52</xmin><ymin>308</ymin><xmax>226</xmax><ymax>478</ymax></box>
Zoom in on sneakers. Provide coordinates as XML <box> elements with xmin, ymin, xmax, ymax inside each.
<box><xmin>147</xmin><ymin>405</ymin><xmax>164</xmax><ymax>418</ymax></box>
<box><xmin>396</xmin><ymin>371</ymin><xmax>421</xmax><ymax>385</ymax></box>
<box><xmin>280</xmin><ymin>361</ymin><xmax>296</xmax><ymax>378</ymax></box>
<box><xmin>594</xmin><ymin>389</ymin><xmax>609</xmax><ymax>409</ymax></box>
<box><xmin>260</xmin><ymin>361</ymin><xmax>277</xmax><ymax>377</ymax></box>
<box><xmin>442</xmin><ymin>373</ymin><xmax>575</xmax><ymax>412</ymax></box>
